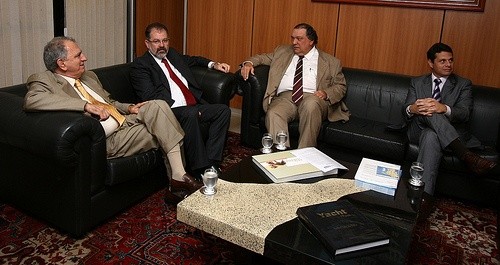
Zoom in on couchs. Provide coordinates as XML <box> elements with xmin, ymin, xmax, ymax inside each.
<box><xmin>235</xmin><ymin>64</ymin><xmax>500</xmax><ymax>208</ymax></box>
<box><xmin>0</xmin><ymin>62</ymin><xmax>236</xmax><ymax>239</ymax></box>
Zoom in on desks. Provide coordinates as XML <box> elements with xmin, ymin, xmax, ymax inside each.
<box><xmin>176</xmin><ymin>145</ymin><xmax>425</xmax><ymax>265</ymax></box>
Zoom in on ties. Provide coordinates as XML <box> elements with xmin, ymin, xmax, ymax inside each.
<box><xmin>74</xmin><ymin>79</ymin><xmax>128</xmax><ymax>132</ymax></box>
<box><xmin>161</xmin><ymin>58</ymin><xmax>196</xmax><ymax>106</ymax></box>
<box><xmin>432</xmin><ymin>79</ymin><xmax>443</xmax><ymax>104</ymax></box>
<box><xmin>291</xmin><ymin>55</ymin><xmax>305</xmax><ymax>106</ymax></box>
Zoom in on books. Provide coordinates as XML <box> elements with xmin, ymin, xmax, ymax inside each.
<box><xmin>252</xmin><ymin>150</ymin><xmax>338</xmax><ymax>183</ymax></box>
<box><xmin>297</xmin><ymin>198</ymin><xmax>390</xmax><ymax>258</ymax></box>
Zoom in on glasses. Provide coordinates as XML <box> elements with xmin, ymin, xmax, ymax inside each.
<box><xmin>146</xmin><ymin>37</ymin><xmax>171</xmax><ymax>44</ymax></box>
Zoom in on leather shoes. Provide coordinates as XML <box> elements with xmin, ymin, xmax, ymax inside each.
<box><xmin>472</xmin><ymin>156</ymin><xmax>497</xmax><ymax>174</ymax></box>
<box><xmin>164</xmin><ymin>187</ymin><xmax>191</xmax><ymax>205</ymax></box>
<box><xmin>171</xmin><ymin>173</ymin><xmax>204</xmax><ymax>192</ymax></box>
<box><xmin>417</xmin><ymin>198</ymin><xmax>434</xmax><ymax>223</ymax></box>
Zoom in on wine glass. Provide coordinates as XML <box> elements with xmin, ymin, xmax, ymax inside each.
<box><xmin>262</xmin><ymin>134</ymin><xmax>273</xmax><ymax>153</ymax></box>
<box><xmin>202</xmin><ymin>168</ymin><xmax>218</xmax><ymax>195</ymax></box>
<box><xmin>409</xmin><ymin>161</ymin><xmax>425</xmax><ymax>187</ymax></box>
<box><xmin>275</xmin><ymin>130</ymin><xmax>288</xmax><ymax>150</ymax></box>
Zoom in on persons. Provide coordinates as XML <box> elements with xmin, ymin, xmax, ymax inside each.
<box><xmin>24</xmin><ymin>36</ymin><xmax>203</xmax><ymax>205</ymax></box>
<box><xmin>240</xmin><ymin>24</ymin><xmax>347</xmax><ymax>149</ymax></box>
<box><xmin>401</xmin><ymin>41</ymin><xmax>496</xmax><ymax>223</ymax></box>
<box><xmin>129</xmin><ymin>24</ymin><xmax>232</xmax><ymax>183</ymax></box>
